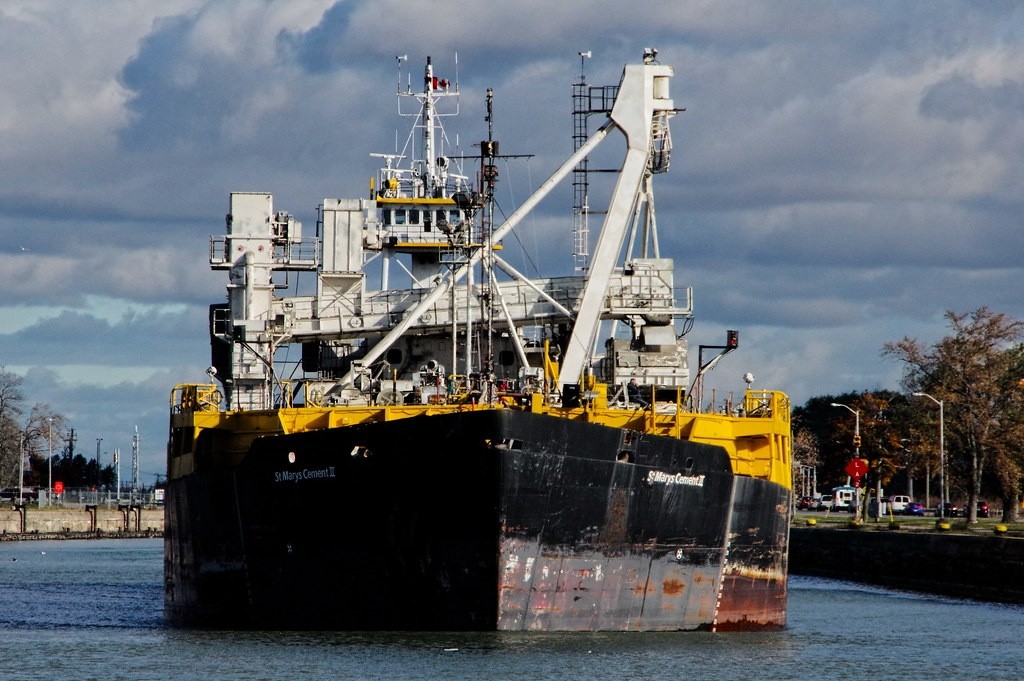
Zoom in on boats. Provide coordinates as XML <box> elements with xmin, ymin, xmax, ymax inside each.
<box><xmin>163</xmin><ymin>51</ymin><xmax>791</xmax><ymax>634</ymax></box>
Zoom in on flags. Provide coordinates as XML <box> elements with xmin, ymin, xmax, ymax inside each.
<box><xmin>433</xmin><ymin>76</ymin><xmax>450</xmax><ymax>91</ymax></box>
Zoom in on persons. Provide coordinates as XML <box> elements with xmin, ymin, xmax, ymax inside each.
<box><xmin>627</xmin><ymin>378</ymin><xmax>651</xmax><ymax>410</ymax></box>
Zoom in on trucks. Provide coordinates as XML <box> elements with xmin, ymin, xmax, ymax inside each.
<box><xmin>830</xmin><ymin>486</ymin><xmax>856</xmax><ymax>513</ymax></box>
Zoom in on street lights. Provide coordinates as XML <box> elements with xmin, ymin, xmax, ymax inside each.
<box><xmin>912</xmin><ymin>393</ymin><xmax>951</xmax><ymax>518</ymax></box>
<box><xmin>49</xmin><ymin>419</ymin><xmax>54</xmax><ymax>506</ymax></box>
<box><xmin>831</xmin><ymin>403</ymin><xmax>860</xmax><ymax>520</ymax></box>
<box><xmin>19</xmin><ymin>430</ymin><xmax>24</xmax><ymax>505</ymax></box>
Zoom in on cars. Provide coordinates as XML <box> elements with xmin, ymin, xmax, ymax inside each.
<box><xmin>903</xmin><ymin>502</ymin><xmax>925</xmax><ymax>516</ymax></box>
<box><xmin>797</xmin><ymin>496</ymin><xmax>814</xmax><ymax>510</ymax></box>
<box><xmin>811</xmin><ymin>497</ymin><xmax>820</xmax><ymax>509</ymax></box>
<box><xmin>935</xmin><ymin>503</ymin><xmax>959</xmax><ymax>518</ymax></box>
<box><xmin>963</xmin><ymin>501</ymin><xmax>988</xmax><ymax>518</ymax></box>
<box><xmin>817</xmin><ymin>495</ymin><xmax>833</xmax><ymax>512</ymax></box>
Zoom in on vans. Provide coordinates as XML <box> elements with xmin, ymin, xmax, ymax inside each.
<box><xmin>889</xmin><ymin>495</ymin><xmax>910</xmax><ymax>512</ymax></box>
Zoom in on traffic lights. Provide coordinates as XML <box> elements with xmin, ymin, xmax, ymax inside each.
<box><xmin>853</xmin><ymin>436</ymin><xmax>861</xmax><ymax>447</ymax></box>
